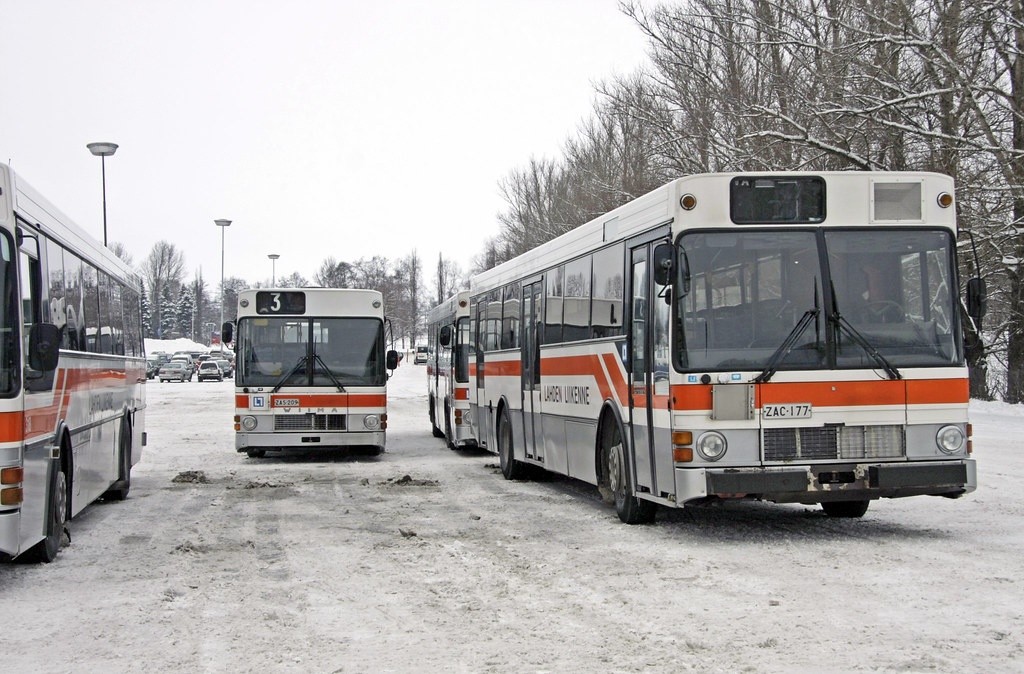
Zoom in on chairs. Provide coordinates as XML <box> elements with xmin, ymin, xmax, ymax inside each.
<box><xmin>685</xmin><ymin>297</ymin><xmax>796</xmax><ymax>350</ymax></box>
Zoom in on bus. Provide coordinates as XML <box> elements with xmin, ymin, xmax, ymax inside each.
<box><xmin>220</xmin><ymin>287</ymin><xmax>399</xmax><ymax>460</ymax></box>
<box><xmin>425</xmin><ymin>291</ymin><xmax>485</xmax><ymax>452</ymax></box>
<box><xmin>468</xmin><ymin>163</ymin><xmax>988</xmax><ymax>524</ymax></box>
<box><xmin>1</xmin><ymin>161</ymin><xmax>151</xmax><ymax>564</ymax></box>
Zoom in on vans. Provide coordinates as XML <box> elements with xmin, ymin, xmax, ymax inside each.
<box><xmin>412</xmin><ymin>346</ymin><xmax>430</xmax><ymax>365</ymax></box>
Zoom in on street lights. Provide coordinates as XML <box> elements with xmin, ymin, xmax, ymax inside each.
<box><xmin>87</xmin><ymin>143</ymin><xmax>119</xmax><ymax>250</ymax></box>
<box><xmin>268</xmin><ymin>253</ymin><xmax>280</xmax><ymax>288</ymax></box>
<box><xmin>213</xmin><ymin>218</ymin><xmax>232</xmax><ymax>362</ymax></box>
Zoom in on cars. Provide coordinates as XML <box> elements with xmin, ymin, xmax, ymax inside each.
<box><xmin>159</xmin><ymin>362</ymin><xmax>192</xmax><ymax>383</ymax></box>
<box><xmin>197</xmin><ymin>362</ymin><xmax>223</xmax><ymax>382</ymax></box>
<box><xmin>145</xmin><ymin>347</ymin><xmax>237</xmax><ymax>379</ymax></box>
